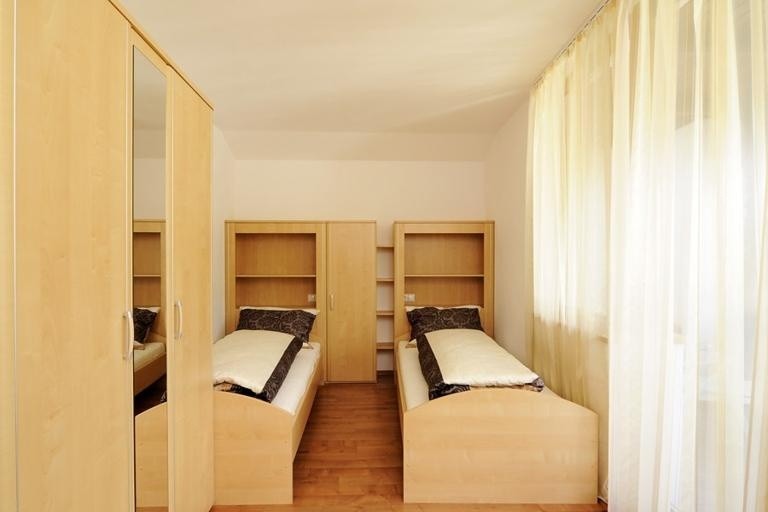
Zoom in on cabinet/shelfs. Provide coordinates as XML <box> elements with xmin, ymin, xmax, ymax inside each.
<box><xmin>132</xmin><ymin>218</ymin><xmax>167</xmax><ymax>343</ymax></box>
<box><xmin>222</xmin><ymin>219</ymin><xmax>326</xmax><ymax>385</ymax></box>
<box><xmin>394</xmin><ymin>219</ymin><xmax>495</xmax><ymax>345</ymax></box>
<box><xmin>376</xmin><ymin>243</ymin><xmax>394</xmax><ymax>350</ymax></box>
<box><xmin>0</xmin><ymin>0</ymin><xmax>214</xmax><ymax>512</ymax></box>
<box><xmin>326</xmin><ymin>220</ymin><xmax>377</xmax><ymax>384</ymax></box>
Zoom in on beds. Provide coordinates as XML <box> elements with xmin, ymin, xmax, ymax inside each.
<box><xmin>135</xmin><ymin>340</ymin><xmax>324</xmax><ymax>507</ymax></box>
<box><xmin>392</xmin><ymin>329</ymin><xmax>599</xmax><ymax>505</ymax></box>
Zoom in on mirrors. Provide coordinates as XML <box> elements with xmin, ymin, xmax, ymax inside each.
<box><xmin>130</xmin><ymin>44</ymin><xmax>171</xmax><ymax>415</ymax></box>
